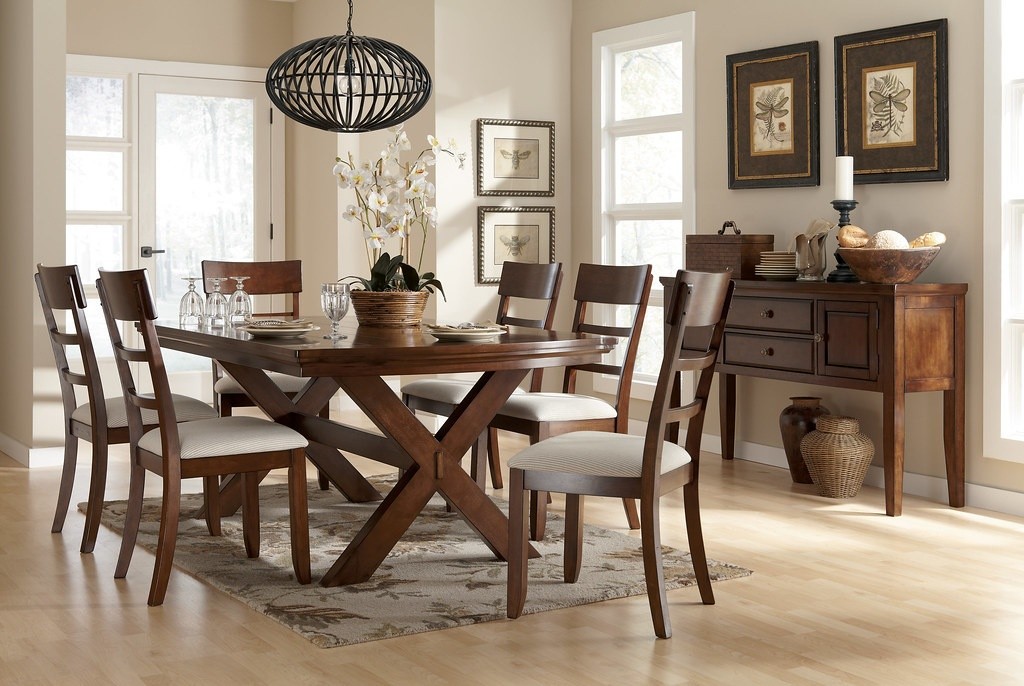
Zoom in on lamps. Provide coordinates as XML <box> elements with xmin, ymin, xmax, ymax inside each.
<box><xmin>265</xmin><ymin>0</ymin><xmax>432</xmax><ymax>133</ymax></box>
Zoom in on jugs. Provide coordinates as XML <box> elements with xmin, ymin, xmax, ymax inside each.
<box><xmin>795</xmin><ymin>232</ymin><xmax>828</xmax><ymax>282</ymax></box>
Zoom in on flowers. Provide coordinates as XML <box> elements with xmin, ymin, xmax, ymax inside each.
<box><xmin>335</xmin><ymin>123</ymin><xmax>466</xmax><ymax>303</ymax></box>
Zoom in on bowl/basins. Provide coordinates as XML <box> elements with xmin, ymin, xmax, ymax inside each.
<box><xmin>837</xmin><ymin>246</ymin><xmax>941</xmax><ymax>285</ymax></box>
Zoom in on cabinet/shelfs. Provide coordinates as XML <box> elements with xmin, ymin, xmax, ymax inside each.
<box><xmin>659</xmin><ymin>276</ymin><xmax>969</xmax><ymax>517</ymax></box>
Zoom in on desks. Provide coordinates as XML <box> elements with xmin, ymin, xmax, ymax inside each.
<box><xmin>134</xmin><ymin>316</ymin><xmax>620</xmax><ymax>588</ymax></box>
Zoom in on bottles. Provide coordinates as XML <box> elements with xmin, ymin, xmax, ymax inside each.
<box><xmin>779</xmin><ymin>396</ymin><xmax>831</xmax><ymax>485</ymax></box>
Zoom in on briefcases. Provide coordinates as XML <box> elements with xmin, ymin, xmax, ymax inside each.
<box><xmin>686</xmin><ymin>221</ymin><xmax>774</xmax><ymax>279</ymax></box>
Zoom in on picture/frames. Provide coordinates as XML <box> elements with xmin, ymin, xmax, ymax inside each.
<box><xmin>834</xmin><ymin>18</ymin><xmax>950</xmax><ymax>184</ymax></box>
<box><xmin>478</xmin><ymin>206</ymin><xmax>555</xmax><ymax>284</ymax></box>
<box><xmin>724</xmin><ymin>40</ymin><xmax>819</xmax><ymax>190</ymax></box>
<box><xmin>477</xmin><ymin>118</ymin><xmax>556</xmax><ymax>198</ymax></box>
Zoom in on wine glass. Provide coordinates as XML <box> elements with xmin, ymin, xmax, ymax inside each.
<box><xmin>179</xmin><ymin>277</ymin><xmax>204</xmax><ymax>325</ymax></box>
<box><xmin>228</xmin><ymin>277</ymin><xmax>252</xmax><ymax>326</ymax></box>
<box><xmin>203</xmin><ymin>277</ymin><xmax>230</xmax><ymax>329</ymax></box>
<box><xmin>320</xmin><ymin>283</ymin><xmax>350</xmax><ymax>340</ymax></box>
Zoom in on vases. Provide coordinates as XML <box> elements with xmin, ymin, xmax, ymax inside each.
<box><xmin>349</xmin><ymin>290</ymin><xmax>430</xmax><ymax>327</ymax></box>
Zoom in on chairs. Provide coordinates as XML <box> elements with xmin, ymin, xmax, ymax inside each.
<box><xmin>32</xmin><ymin>259</ymin><xmax>330</xmax><ymax>607</ymax></box>
<box><xmin>402</xmin><ymin>260</ymin><xmax>734</xmax><ymax>639</ymax></box>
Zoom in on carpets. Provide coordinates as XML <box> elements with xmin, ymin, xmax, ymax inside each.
<box><xmin>77</xmin><ymin>471</ymin><xmax>754</xmax><ymax>648</ymax></box>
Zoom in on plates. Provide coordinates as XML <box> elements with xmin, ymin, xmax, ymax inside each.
<box><xmin>432</xmin><ymin>328</ymin><xmax>508</xmax><ymax>342</ymax></box>
<box><xmin>235</xmin><ymin>325</ymin><xmax>321</xmax><ymax>337</ymax></box>
<box><xmin>754</xmin><ymin>251</ymin><xmax>798</xmax><ymax>281</ymax></box>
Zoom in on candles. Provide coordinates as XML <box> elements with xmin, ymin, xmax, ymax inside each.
<box><xmin>836</xmin><ymin>157</ymin><xmax>854</xmax><ymax>200</ymax></box>
